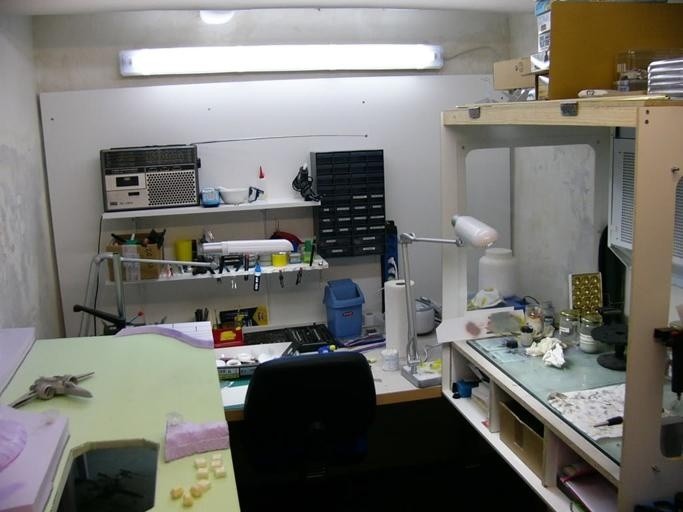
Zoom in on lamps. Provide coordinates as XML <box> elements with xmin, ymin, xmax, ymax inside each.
<box><xmin>117</xmin><ymin>45</ymin><xmax>444</xmax><ymax>78</ymax></box>
<box><xmin>78</xmin><ymin>239</ymin><xmax>294</xmax><ymax>337</ymax></box>
<box><xmin>398</xmin><ymin>214</ymin><xmax>498</xmax><ymax>389</ymax></box>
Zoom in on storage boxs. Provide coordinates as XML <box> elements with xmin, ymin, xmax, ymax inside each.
<box><xmin>493</xmin><ymin>57</ymin><xmax>536</xmax><ymax>91</ymax></box>
<box><xmin>498</xmin><ymin>399</ymin><xmax>543</xmax><ymax>479</ymax></box>
<box><xmin>106</xmin><ymin>232</ymin><xmax>164</xmax><ymax>281</ymax></box>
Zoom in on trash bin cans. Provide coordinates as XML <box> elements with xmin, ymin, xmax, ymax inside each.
<box><xmin>322</xmin><ymin>278</ymin><xmax>366</xmax><ymax>338</ymax></box>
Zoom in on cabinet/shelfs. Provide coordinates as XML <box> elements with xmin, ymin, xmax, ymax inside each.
<box><xmin>104</xmin><ymin>327</ymin><xmax>441</xmax><ymax>423</ymax></box>
<box><xmin>441</xmin><ymin>98</ymin><xmax>683</xmax><ymax>511</ymax></box>
<box><xmin>101</xmin><ymin>197</ymin><xmax>330</xmax><ymax>287</ymax></box>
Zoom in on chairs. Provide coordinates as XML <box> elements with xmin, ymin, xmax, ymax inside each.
<box><xmin>229</xmin><ymin>352</ymin><xmax>386</xmax><ymax>511</ymax></box>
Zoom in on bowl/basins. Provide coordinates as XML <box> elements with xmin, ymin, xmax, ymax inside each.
<box><xmin>217</xmin><ymin>188</ymin><xmax>251</xmax><ymax>204</ymax></box>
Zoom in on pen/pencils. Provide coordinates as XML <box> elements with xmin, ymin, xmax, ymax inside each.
<box><xmin>109</xmin><ymin>231</ymin><xmax>136</xmax><ymax>245</ymax></box>
<box><xmin>308</xmin><ymin>236</ymin><xmax>319</xmax><ymax>265</ymax></box>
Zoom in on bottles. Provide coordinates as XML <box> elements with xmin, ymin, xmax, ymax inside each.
<box><xmin>479</xmin><ymin>248</ymin><xmax>518</xmax><ymax>299</ymax></box>
<box><xmin>520</xmin><ymin>301</ymin><xmax>555</xmax><ymax>348</ymax></box>
<box><xmin>558</xmin><ymin>310</ymin><xmax>602</xmax><ymax>353</ymax></box>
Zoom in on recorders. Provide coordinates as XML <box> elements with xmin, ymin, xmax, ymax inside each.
<box><xmin>100</xmin><ymin>144</ymin><xmax>202</xmax><ymax>213</ymax></box>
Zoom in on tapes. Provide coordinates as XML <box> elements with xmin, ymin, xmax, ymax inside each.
<box><xmin>239</xmin><ymin>354</ymin><xmax>255</xmax><ymax>363</ymax></box>
<box><xmin>272</xmin><ymin>253</ymin><xmax>287</xmax><ymax>266</ymax></box>
<box><xmin>215</xmin><ymin>359</ymin><xmax>239</xmax><ymax>368</ymax></box>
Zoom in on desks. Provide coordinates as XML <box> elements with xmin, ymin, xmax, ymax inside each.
<box><xmin>0</xmin><ymin>333</ymin><xmax>242</xmax><ymax>512</ymax></box>
